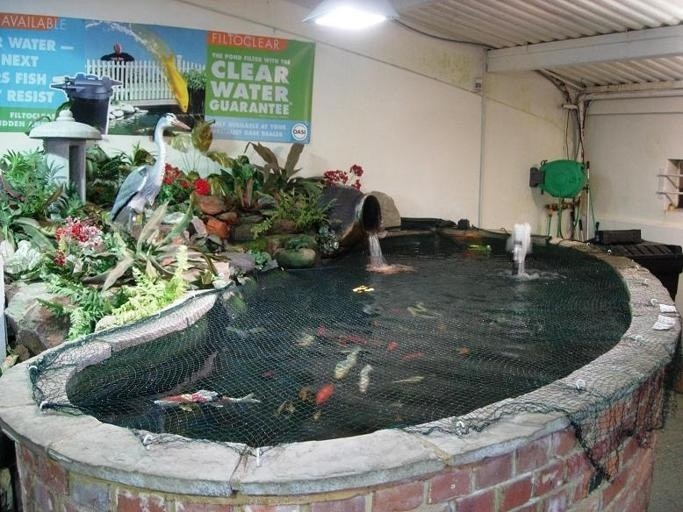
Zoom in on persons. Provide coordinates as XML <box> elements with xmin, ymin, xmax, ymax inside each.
<box><xmin>100</xmin><ymin>43</ymin><xmax>134</xmax><ymax>88</ymax></box>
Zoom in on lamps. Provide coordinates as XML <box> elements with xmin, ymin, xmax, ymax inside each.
<box><xmin>300</xmin><ymin>0</ymin><xmax>401</xmax><ymax>33</ymax></box>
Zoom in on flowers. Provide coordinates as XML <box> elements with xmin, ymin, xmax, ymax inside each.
<box><xmin>45</xmin><ymin>213</ymin><xmax>111</xmax><ymax>271</ymax></box>
<box><xmin>323</xmin><ymin>161</ymin><xmax>365</xmax><ymax>192</ymax></box>
<box><xmin>164</xmin><ymin>164</ymin><xmax>210</xmax><ymax>197</ymax></box>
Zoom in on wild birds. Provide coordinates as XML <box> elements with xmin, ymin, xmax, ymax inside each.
<box><xmin>109</xmin><ymin>112</ymin><xmax>191</xmax><ymax>233</ymax></box>
